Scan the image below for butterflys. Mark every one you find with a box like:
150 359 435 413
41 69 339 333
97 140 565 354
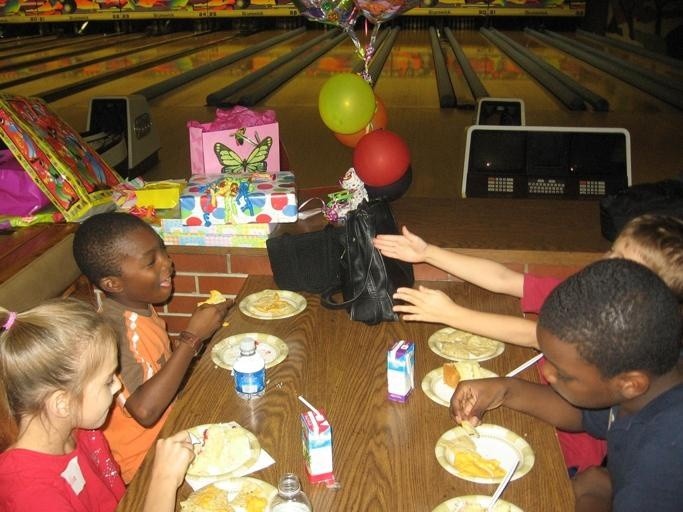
214 136 272 173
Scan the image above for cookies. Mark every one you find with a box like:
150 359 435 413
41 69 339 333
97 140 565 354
461 419 481 438
196 289 226 308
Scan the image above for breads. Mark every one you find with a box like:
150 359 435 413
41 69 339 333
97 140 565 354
187 425 250 477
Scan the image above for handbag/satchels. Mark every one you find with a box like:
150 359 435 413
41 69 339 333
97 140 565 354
343 197 416 327
189 119 282 177
265 225 343 295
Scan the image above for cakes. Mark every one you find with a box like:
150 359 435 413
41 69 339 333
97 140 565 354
444 435 509 478
443 360 480 389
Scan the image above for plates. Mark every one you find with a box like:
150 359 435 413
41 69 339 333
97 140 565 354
239 290 307 320
428 326 506 362
434 423 535 485
421 366 501 410
210 332 289 370
180 423 273 511
430 494 522 512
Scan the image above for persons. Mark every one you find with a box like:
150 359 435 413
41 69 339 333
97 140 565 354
73 212 234 486
0 297 196 512
371 214 683 353
449 258 683 512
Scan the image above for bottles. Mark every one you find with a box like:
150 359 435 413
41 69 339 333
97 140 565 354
233 337 266 422
269 474 310 511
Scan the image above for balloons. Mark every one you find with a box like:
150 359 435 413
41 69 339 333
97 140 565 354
299 0 412 187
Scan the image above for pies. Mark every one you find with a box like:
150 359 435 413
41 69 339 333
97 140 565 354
433 330 497 359
179 479 267 512
247 292 295 316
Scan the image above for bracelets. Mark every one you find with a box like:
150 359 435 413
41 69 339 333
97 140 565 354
175 330 205 356
